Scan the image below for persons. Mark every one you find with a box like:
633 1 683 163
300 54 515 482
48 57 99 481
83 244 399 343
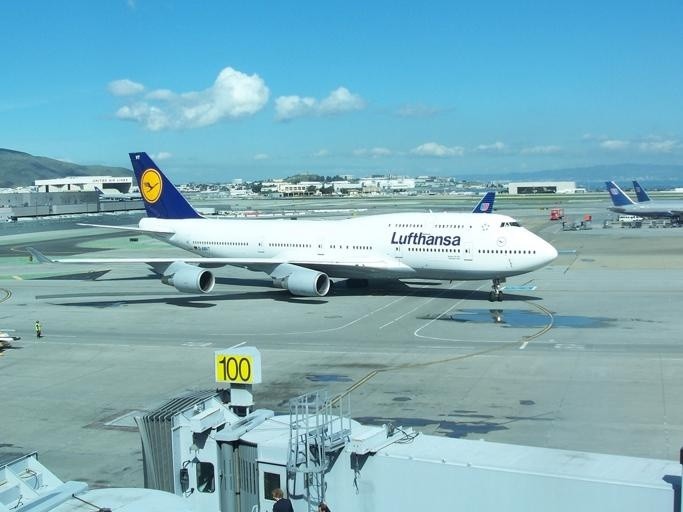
317 501 331 512
271 488 294 512
35 320 41 338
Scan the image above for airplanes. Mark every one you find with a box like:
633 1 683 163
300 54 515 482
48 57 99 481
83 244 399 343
26 153 559 302
604 179 683 226
93 186 142 201
